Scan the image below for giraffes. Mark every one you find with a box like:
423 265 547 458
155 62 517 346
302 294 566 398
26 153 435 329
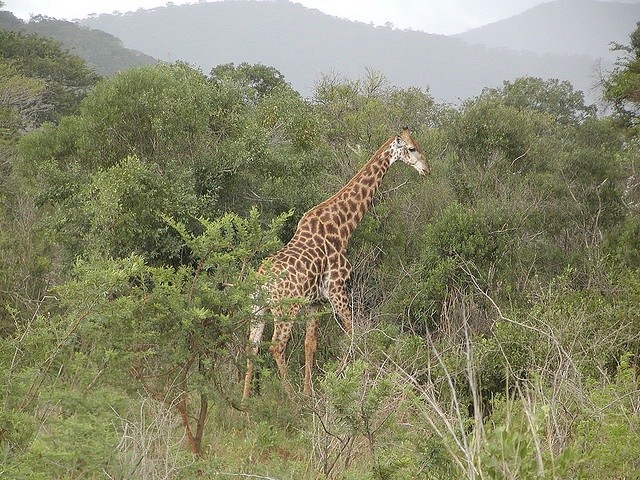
241 124 432 410
